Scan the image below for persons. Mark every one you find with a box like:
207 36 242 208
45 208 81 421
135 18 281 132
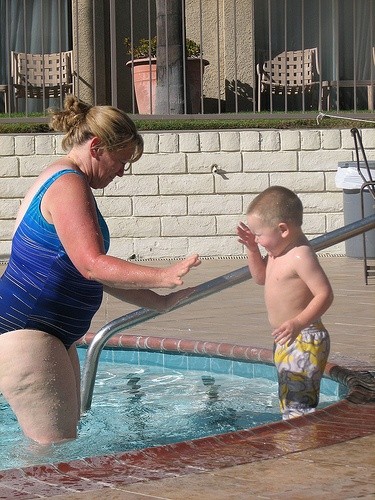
236 186 335 422
0 95 201 445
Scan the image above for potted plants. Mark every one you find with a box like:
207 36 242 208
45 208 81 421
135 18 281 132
124 35 210 114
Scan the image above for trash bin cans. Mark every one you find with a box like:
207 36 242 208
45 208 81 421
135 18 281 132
336 160 375 259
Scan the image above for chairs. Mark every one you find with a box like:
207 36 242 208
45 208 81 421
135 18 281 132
0 50 74 114
255 48 331 111
